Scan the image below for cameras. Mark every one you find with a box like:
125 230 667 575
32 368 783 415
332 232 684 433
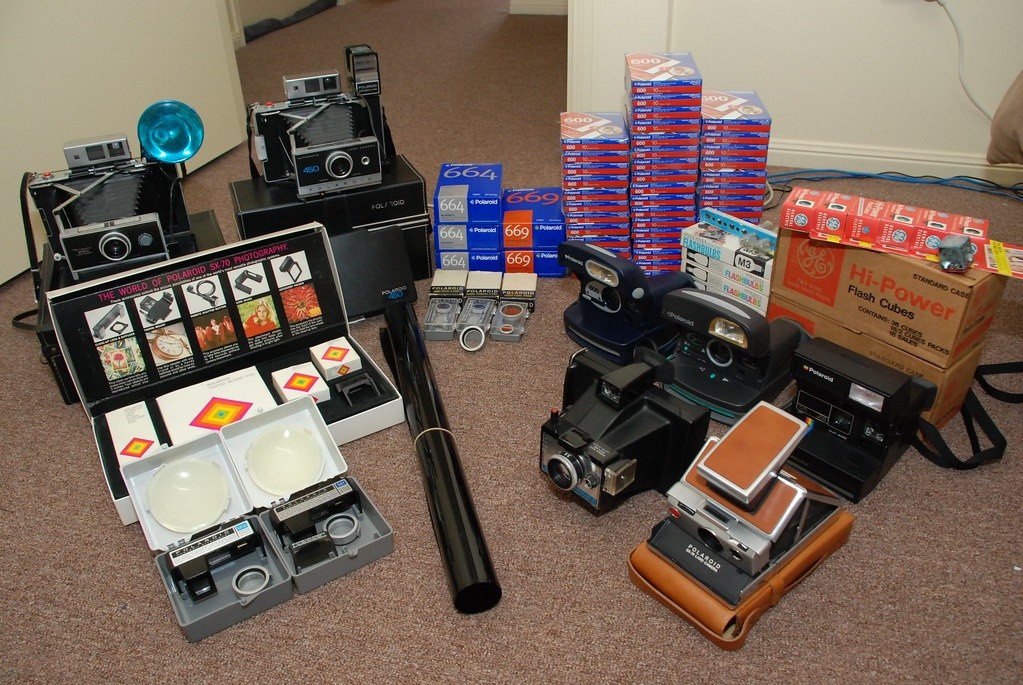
28 99 204 287
537 241 937 653
253 44 395 199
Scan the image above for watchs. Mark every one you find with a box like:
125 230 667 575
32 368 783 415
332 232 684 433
151 328 192 360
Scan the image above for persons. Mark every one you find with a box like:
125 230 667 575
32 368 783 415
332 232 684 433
244 300 278 338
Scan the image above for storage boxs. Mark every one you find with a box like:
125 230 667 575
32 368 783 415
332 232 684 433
44 221 405 525
433 224 500 253
500 249 566 278
778 186 989 263
309 336 363 381
155 365 278 446
428 268 537 315
499 189 565 250
766 292 987 443
270 363 331 405
435 250 501 272
105 400 160 466
770 226 1008 371
559 52 777 317
433 163 502 224
